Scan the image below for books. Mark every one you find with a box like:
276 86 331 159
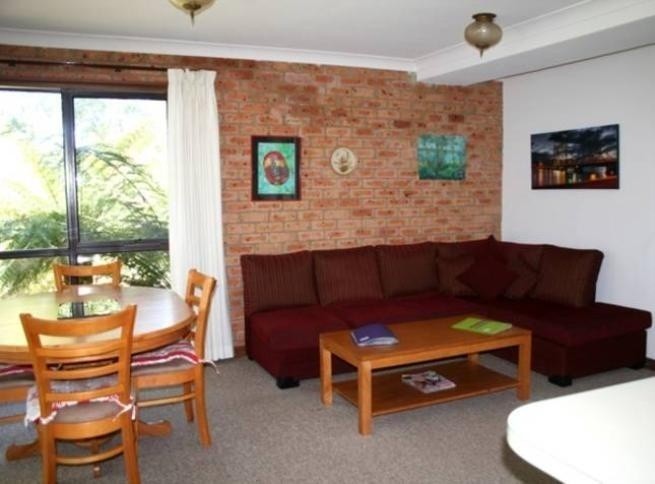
450 316 513 336
351 323 400 347
400 369 457 395
57 298 123 319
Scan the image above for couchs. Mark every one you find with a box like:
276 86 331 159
241 234 653 391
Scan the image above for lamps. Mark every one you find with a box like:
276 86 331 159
464 10 504 56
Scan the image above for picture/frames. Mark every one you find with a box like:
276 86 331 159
249 134 302 203
